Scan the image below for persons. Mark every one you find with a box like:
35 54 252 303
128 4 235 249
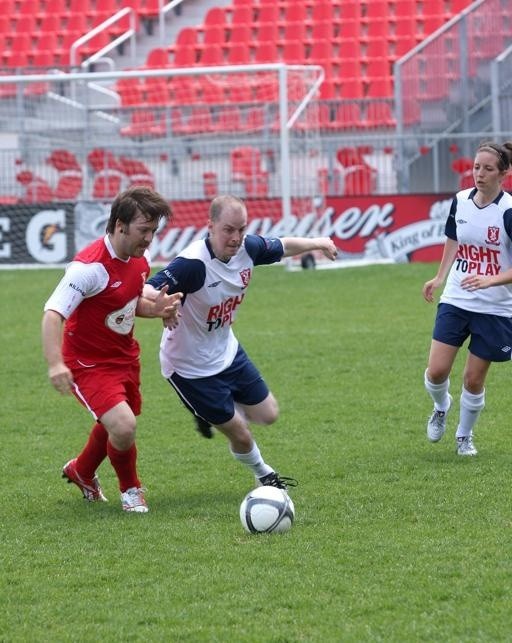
41 187 185 514
146 193 340 488
424 142 512 456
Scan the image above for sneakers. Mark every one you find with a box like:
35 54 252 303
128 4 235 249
456 425 477 456
119 487 148 513
255 466 286 489
195 414 213 439
427 395 454 442
62 459 109 502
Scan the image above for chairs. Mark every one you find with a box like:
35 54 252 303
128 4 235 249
394 1 416 18
19 0 41 20
424 56 449 78
255 21 278 42
0 14 13 38
338 39 361 58
32 50 54 66
365 38 391 58
202 7 228 24
88 30 107 48
330 105 361 128
287 75 303 103
229 23 251 43
309 60 332 79
422 15 444 37
69 0 91 18
365 78 393 98
13 33 32 51
451 1 474 17
8 51 28 67
0 1 17 21
254 41 278 63
361 103 392 128
170 77 198 107
308 40 333 59
421 76 449 101
400 78 420 99
272 106 304 131
96 0 116 16
42 15 61 36
254 72 278 101
189 109 210 132
119 1 142 17
37 33 57 51
45 0 66 20
389 98 423 128
67 13 87 32
423 2 446 16
217 107 242 131
116 79 144 104
16 17 36 39
282 39 306 64
0 33 7 51
201 45 226 66
175 46 195 66
367 17 390 38
245 107 267 133
450 57 475 77
63 34 82 48
311 4 335 20
398 57 419 77
394 37 417 57
306 104 329 129
337 58 361 77
158 109 184 134
228 43 251 63
131 111 155 135
365 57 391 77
140 1 168 17
336 17 361 38
394 16 415 36
146 48 169 67
311 18 335 39
204 24 226 46
176 28 198 45
60 50 80 65
320 80 336 101
364 3 390 19
338 3 362 19
231 5 254 23
338 81 364 99
144 77 169 106
284 5 307 21
199 76 224 104
285 21 306 39
228 73 253 101
258 5 280 22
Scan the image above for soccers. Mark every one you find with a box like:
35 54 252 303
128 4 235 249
239 485 295 535
